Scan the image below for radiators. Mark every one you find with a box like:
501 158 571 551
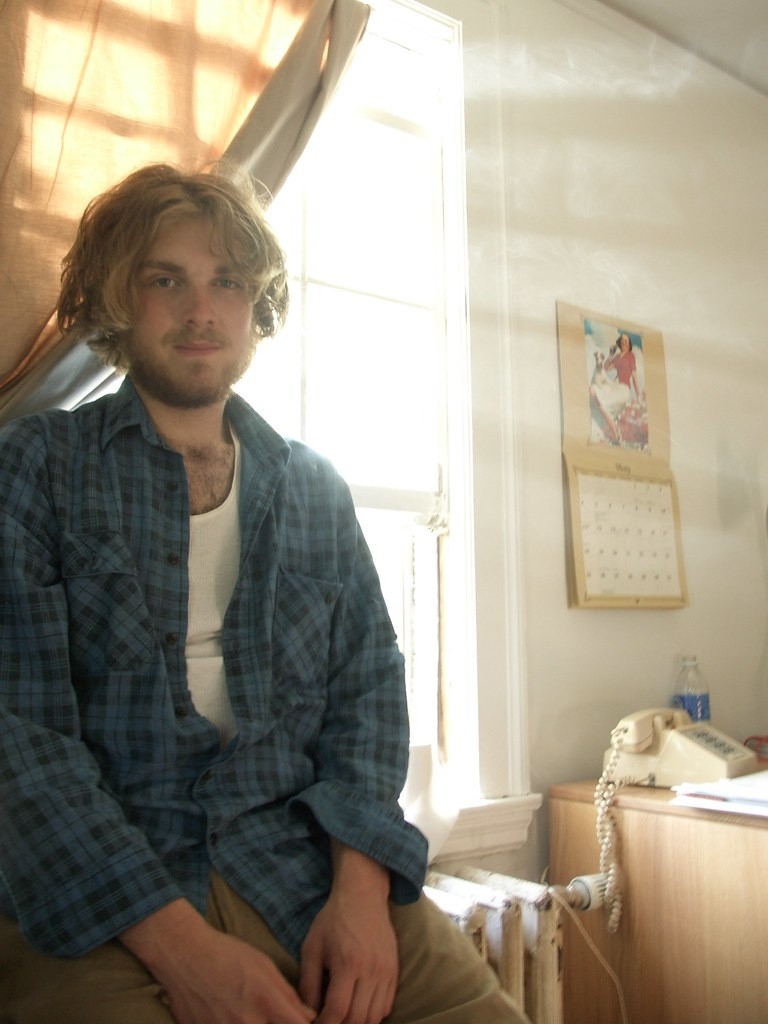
422 865 606 1024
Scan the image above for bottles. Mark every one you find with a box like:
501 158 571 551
674 654 712 724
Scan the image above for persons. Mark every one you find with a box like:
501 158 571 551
0 163 535 1024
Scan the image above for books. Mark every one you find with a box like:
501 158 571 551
670 767 767 820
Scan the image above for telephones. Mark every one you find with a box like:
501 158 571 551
601 706 758 789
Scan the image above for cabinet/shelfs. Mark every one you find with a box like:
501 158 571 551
547 762 768 1024
